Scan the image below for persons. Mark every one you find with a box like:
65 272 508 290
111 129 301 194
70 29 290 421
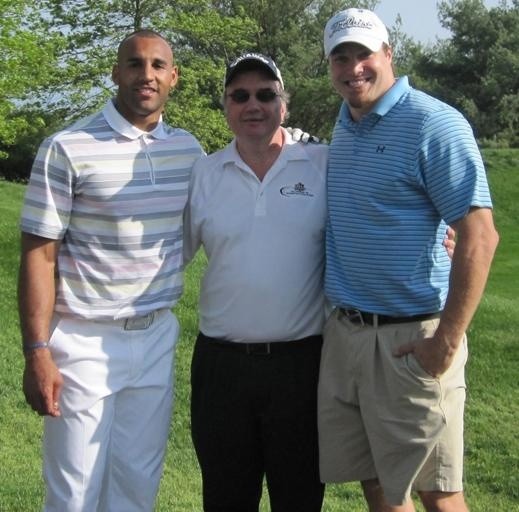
183 53 457 511
318 6 499 512
17 30 319 512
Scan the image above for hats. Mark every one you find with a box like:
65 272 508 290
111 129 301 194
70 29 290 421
322 7 390 64
224 51 285 91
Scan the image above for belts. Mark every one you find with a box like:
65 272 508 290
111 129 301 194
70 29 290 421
199 329 325 365
333 299 450 334
51 305 174 333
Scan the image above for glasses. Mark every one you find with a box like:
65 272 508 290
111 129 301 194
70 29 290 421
224 86 282 105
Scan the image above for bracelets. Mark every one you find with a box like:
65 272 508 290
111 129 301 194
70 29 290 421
22 342 48 352
